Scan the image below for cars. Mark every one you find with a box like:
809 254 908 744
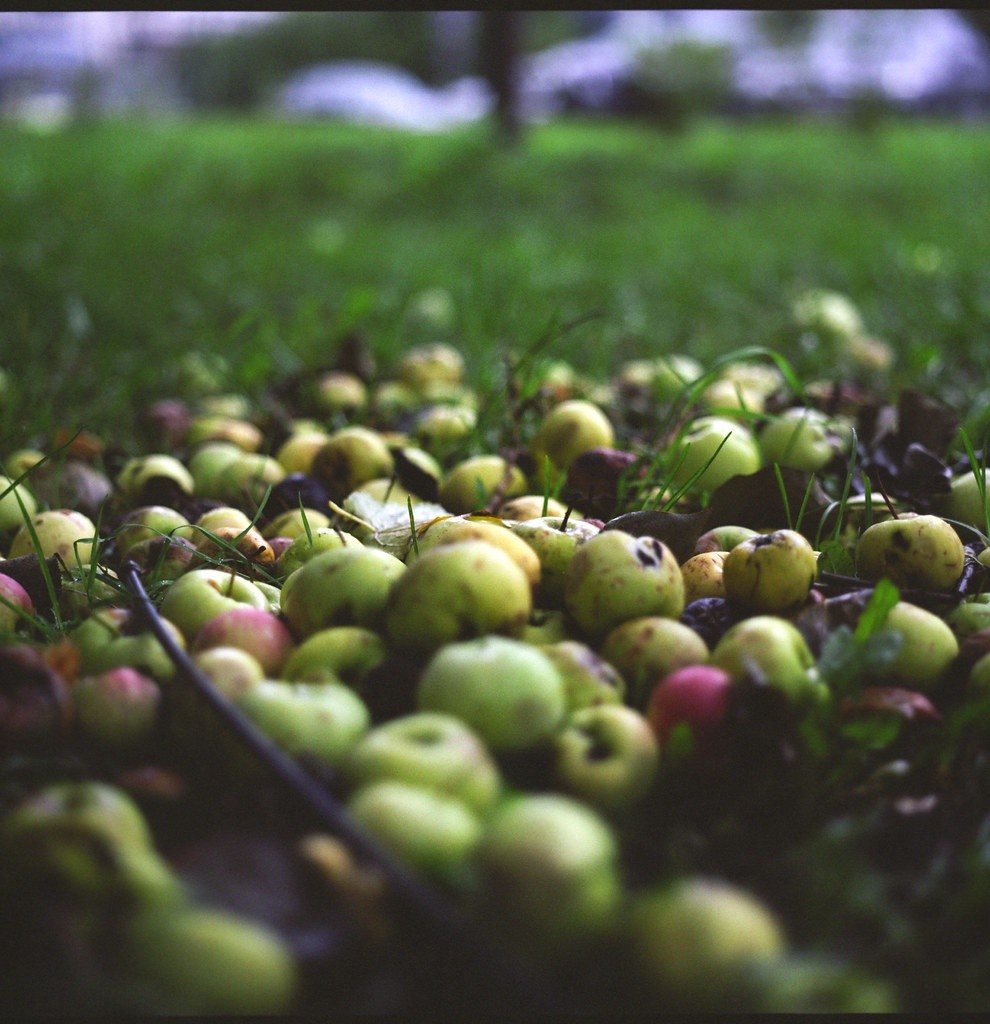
452 10 988 130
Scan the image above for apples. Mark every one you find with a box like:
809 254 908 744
0 285 990 1024
